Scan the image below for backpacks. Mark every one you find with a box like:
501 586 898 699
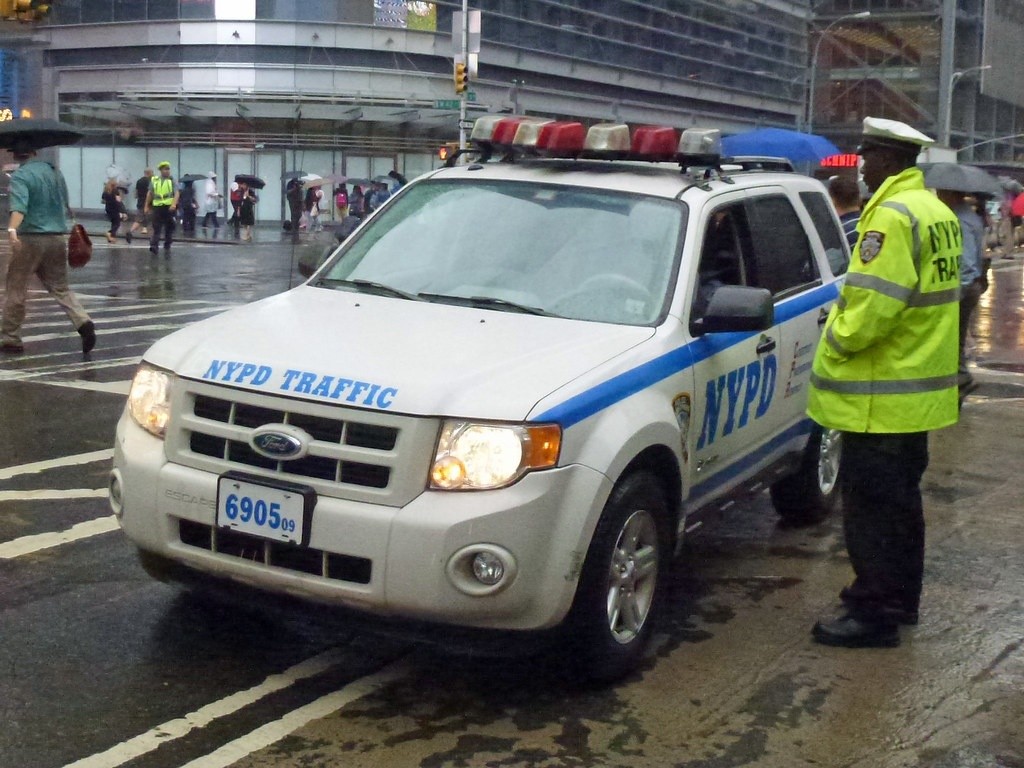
336 193 348 208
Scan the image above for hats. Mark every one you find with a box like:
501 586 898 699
855 116 936 156
157 161 170 169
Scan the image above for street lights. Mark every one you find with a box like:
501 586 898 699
805 11 872 137
943 64 992 149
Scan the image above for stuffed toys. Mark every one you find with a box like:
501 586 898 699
106 162 132 221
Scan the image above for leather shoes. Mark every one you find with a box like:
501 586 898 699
839 585 920 626
811 611 901 648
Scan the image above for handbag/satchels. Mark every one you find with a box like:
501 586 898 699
309 202 320 217
67 225 94 270
980 257 993 294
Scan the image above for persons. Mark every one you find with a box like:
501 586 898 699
805 116 960 644
936 189 984 409
0 139 97 352
825 175 861 251
103 159 405 254
968 192 1024 262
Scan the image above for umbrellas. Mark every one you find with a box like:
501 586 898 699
304 178 334 188
373 175 400 189
721 124 842 162
280 170 308 179
327 175 349 183
301 173 323 179
0 117 83 148
345 178 372 186
234 174 265 189
178 174 208 183
998 176 1023 193
389 170 408 184
914 161 1001 195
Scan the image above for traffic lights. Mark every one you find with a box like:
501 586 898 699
438 143 453 161
454 62 469 94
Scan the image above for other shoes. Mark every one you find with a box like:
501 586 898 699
201 226 223 229
0 342 24 355
77 321 96 353
957 379 978 409
315 225 323 232
184 233 197 238
105 231 117 244
125 229 133 244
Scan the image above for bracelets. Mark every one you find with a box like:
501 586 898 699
8 228 16 231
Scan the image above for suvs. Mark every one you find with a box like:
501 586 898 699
106 112 858 684
